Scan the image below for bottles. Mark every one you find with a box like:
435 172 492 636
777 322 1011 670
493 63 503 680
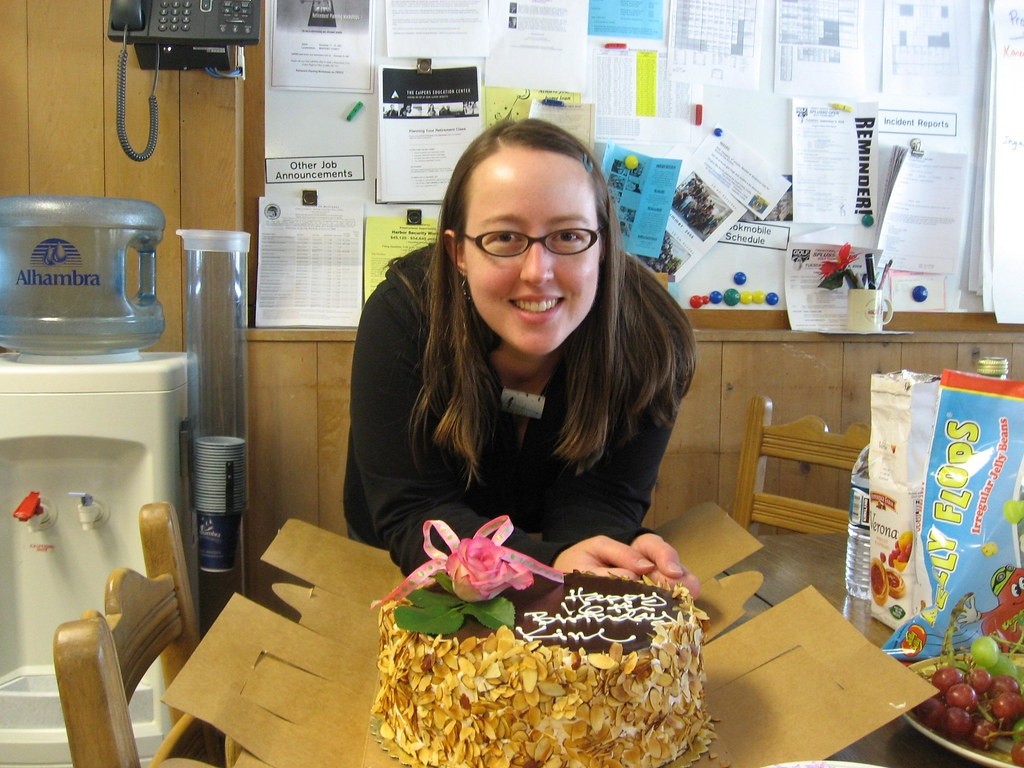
843 444 871 636
0 192 167 354
976 356 1009 380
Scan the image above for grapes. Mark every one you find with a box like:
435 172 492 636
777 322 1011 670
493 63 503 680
912 635 1024 766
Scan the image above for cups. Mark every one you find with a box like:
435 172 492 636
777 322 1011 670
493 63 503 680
192 436 250 573
846 288 894 332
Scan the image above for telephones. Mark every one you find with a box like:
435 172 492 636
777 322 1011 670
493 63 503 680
107 0 260 46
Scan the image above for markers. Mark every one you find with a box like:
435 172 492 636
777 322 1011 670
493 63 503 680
865 253 876 289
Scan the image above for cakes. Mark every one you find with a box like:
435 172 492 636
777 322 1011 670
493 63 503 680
370 569 722 768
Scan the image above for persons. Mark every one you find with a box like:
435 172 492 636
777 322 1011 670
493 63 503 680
672 177 722 236
637 231 678 274
343 119 697 601
387 101 477 118
766 192 793 221
607 159 644 236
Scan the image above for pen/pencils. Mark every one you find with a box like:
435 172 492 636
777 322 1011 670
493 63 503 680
843 258 892 290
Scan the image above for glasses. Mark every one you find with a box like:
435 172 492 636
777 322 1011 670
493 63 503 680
464 227 604 258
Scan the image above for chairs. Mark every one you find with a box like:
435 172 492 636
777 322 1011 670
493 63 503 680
52 497 224 768
729 397 877 539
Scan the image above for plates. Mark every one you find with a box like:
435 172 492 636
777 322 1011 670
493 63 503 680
902 652 1024 768
